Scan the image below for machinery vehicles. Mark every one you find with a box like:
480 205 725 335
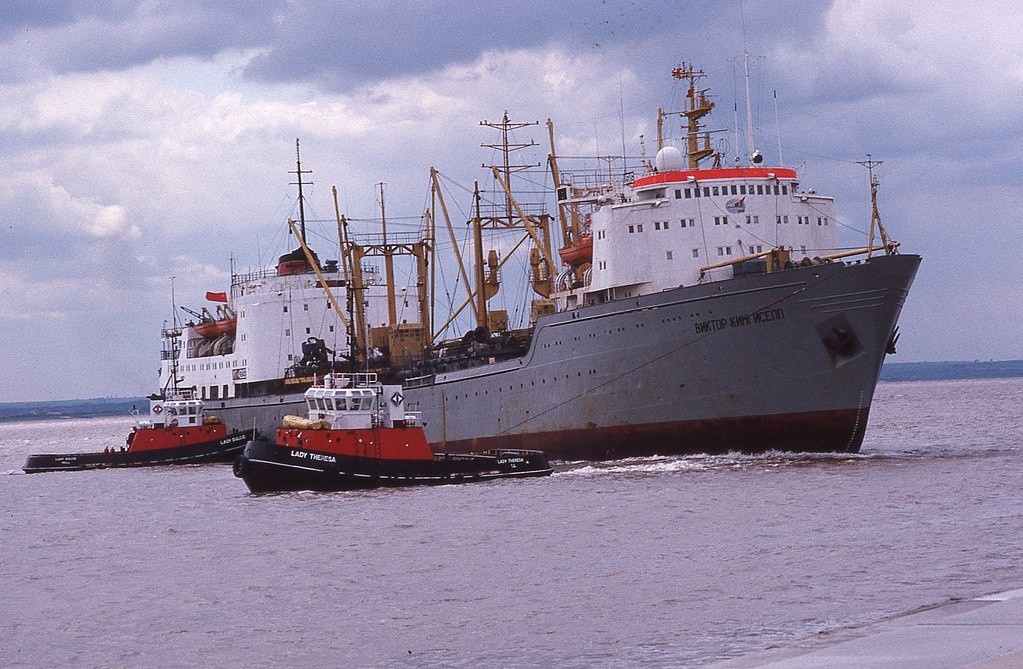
277 119 591 392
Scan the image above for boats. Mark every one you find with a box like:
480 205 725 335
20 389 262 474
230 365 555 496
142 0 923 461
